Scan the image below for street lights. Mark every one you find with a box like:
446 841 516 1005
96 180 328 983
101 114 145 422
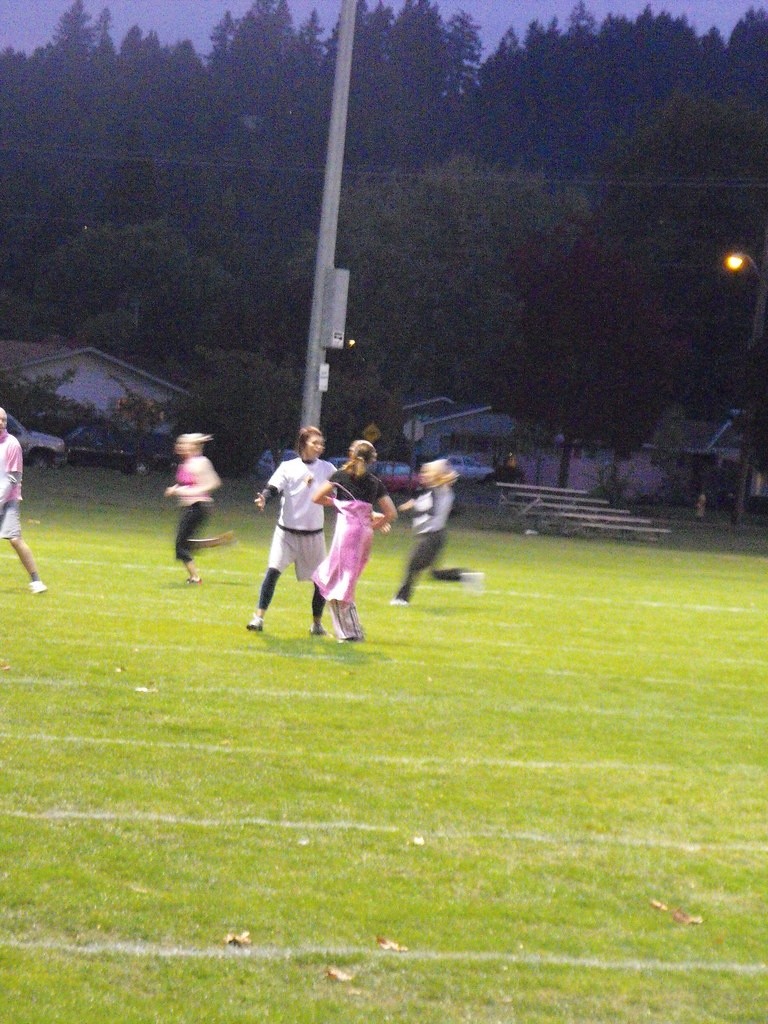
726 250 767 526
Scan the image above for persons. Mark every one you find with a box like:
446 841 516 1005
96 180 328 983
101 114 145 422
0 407 47 595
246 427 390 636
390 460 485 607
311 440 398 642
165 433 237 583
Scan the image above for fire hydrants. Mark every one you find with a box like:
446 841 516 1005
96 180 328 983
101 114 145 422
693 494 709 521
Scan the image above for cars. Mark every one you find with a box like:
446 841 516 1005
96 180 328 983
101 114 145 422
435 454 496 484
375 460 422 492
257 449 300 482
7 414 67 471
66 422 181 477
327 457 350 470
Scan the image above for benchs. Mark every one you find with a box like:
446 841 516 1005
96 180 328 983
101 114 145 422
495 482 672 543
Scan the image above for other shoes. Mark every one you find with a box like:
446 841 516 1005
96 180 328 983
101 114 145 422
29 580 47 594
246 613 264 631
310 623 333 637
390 598 409 606
186 577 202 584
